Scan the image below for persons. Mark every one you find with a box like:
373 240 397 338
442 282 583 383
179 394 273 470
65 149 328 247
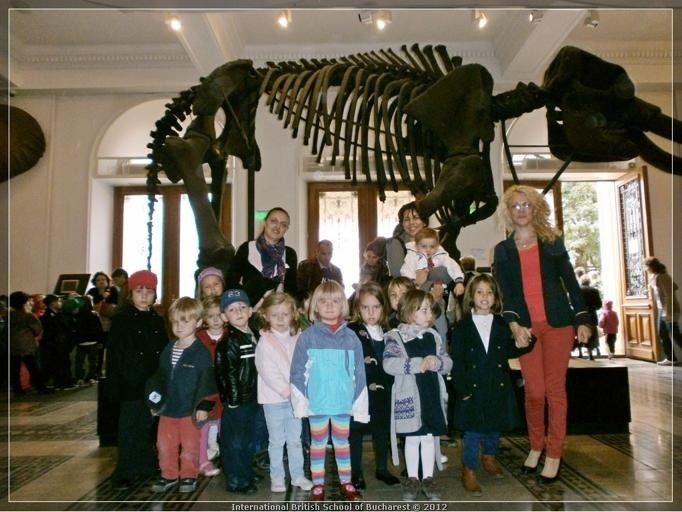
0 184 601 502
642 257 682 366
598 300 619 358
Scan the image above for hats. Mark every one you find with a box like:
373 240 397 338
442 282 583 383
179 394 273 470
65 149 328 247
10 291 94 313
198 266 224 285
220 288 251 313
127 270 157 292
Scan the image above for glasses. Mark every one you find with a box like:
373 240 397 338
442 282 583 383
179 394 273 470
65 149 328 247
508 201 532 210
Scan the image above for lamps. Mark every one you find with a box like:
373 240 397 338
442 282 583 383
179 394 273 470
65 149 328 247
165 13 181 31
278 11 291 30
376 11 391 30
584 11 600 30
472 11 488 29
529 10 544 23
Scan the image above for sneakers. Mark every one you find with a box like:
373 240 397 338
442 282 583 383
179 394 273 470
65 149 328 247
657 358 677 365
23 379 101 395
